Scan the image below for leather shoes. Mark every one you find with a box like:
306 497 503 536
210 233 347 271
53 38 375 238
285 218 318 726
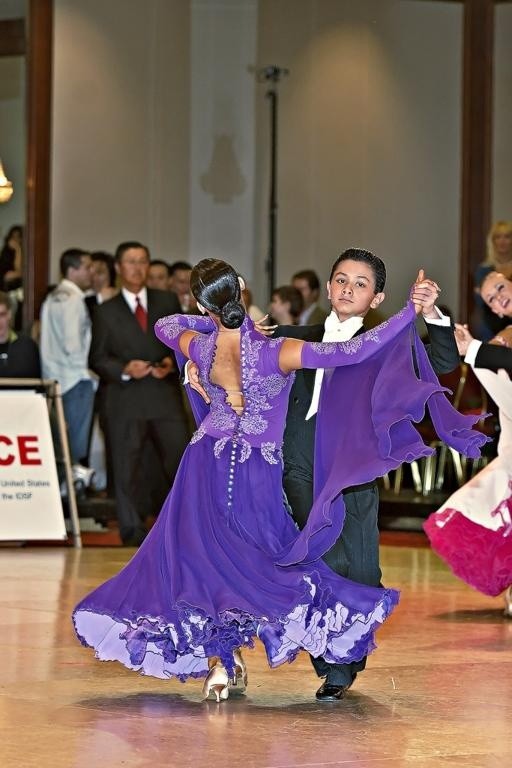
315 664 356 701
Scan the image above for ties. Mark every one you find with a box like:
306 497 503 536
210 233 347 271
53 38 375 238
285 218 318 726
134 297 147 334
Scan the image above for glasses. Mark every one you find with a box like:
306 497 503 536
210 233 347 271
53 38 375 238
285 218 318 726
119 258 151 267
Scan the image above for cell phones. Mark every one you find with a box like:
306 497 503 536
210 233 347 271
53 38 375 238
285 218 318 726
153 361 165 370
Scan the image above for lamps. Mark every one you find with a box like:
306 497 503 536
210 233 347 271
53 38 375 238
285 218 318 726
197 130 246 203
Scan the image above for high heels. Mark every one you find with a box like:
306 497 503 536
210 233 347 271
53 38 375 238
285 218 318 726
228 650 248 694
202 663 229 704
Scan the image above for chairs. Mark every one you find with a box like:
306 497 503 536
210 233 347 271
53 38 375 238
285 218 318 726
370 356 493 499
